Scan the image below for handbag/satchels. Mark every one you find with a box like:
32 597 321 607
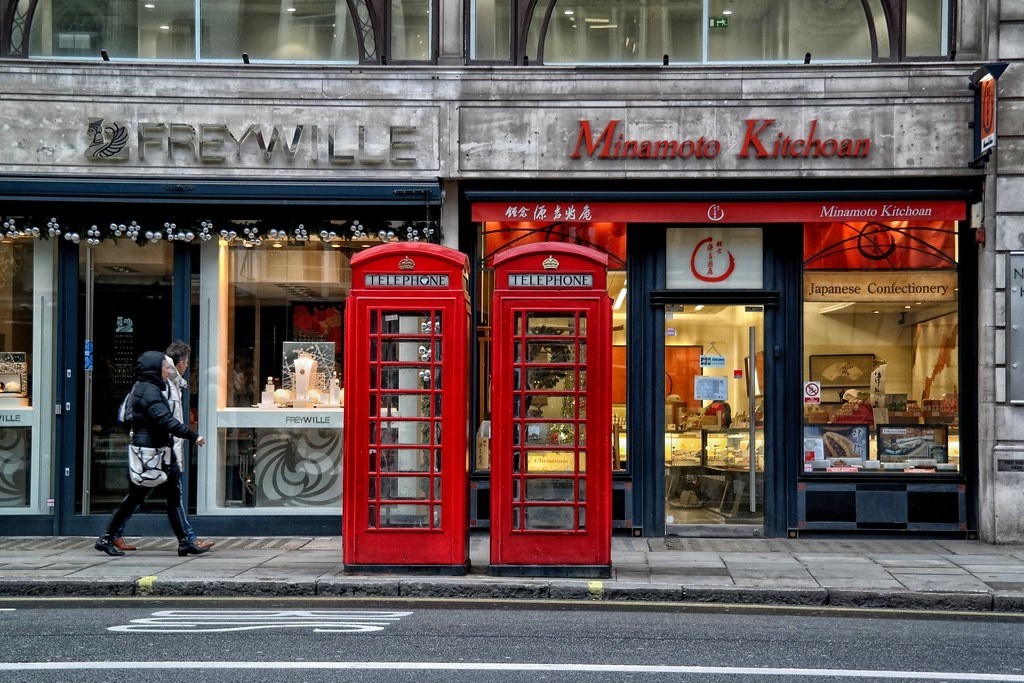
128 444 172 487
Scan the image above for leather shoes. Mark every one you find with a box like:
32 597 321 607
193 539 215 548
112 536 136 550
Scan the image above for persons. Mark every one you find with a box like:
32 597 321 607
704 396 732 429
233 358 253 407
889 434 899 451
96 338 214 557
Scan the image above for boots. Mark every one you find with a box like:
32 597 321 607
167 507 210 556
95 508 128 556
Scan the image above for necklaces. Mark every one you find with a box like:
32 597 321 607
296 357 311 374
